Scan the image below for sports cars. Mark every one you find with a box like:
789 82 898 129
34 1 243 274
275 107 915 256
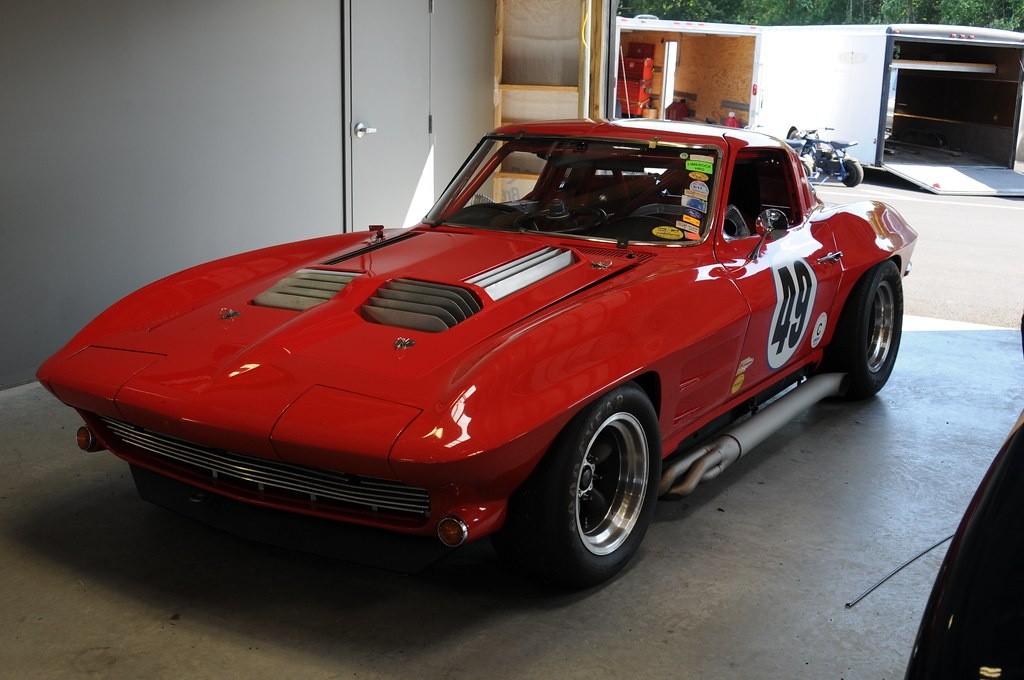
36 117 920 595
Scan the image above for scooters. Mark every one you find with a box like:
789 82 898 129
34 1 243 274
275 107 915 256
740 122 830 187
787 124 864 187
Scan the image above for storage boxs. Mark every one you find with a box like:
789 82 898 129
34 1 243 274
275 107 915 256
616 79 652 115
617 56 654 80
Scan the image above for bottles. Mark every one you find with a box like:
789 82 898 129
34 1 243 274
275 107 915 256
665 97 689 121
725 111 741 128
